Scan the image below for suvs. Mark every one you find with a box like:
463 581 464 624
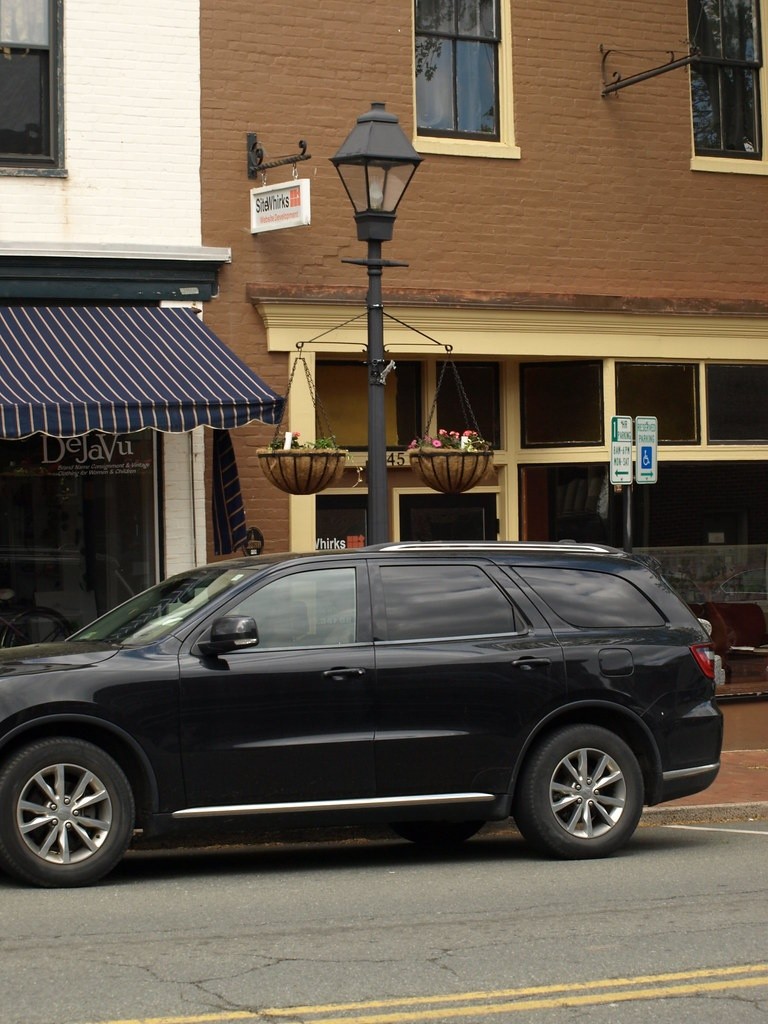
2 536 729 885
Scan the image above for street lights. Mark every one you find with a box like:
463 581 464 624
330 95 426 544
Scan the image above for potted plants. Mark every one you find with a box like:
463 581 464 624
406 448 495 498
255 447 347 497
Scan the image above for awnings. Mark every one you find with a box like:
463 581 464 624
0 303 288 443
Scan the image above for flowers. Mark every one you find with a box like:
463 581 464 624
266 430 342 451
406 429 491 451
